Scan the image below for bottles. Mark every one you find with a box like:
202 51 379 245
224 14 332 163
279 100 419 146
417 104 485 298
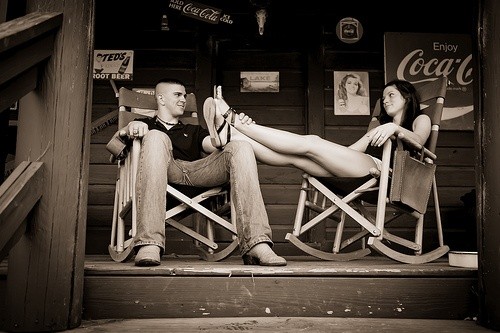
118 55 131 74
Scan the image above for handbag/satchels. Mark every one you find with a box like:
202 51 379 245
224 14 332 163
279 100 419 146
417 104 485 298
393 131 436 216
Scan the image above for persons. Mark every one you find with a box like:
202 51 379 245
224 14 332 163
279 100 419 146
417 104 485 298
112 74 288 267
336 74 369 115
202 79 432 205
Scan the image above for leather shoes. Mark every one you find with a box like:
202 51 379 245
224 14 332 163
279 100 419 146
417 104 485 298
243 242 287 265
133 245 161 266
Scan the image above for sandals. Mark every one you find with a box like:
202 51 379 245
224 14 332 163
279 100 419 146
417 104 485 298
213 85 237 125
203 96 231 148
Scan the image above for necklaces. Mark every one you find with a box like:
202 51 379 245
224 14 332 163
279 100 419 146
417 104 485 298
156 115 179 126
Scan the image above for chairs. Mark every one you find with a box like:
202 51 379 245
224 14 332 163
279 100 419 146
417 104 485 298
285 77 450 264
109 87 239 263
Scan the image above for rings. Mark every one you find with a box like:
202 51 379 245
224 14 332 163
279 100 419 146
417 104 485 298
381 135 385 138
133 129 139 134
